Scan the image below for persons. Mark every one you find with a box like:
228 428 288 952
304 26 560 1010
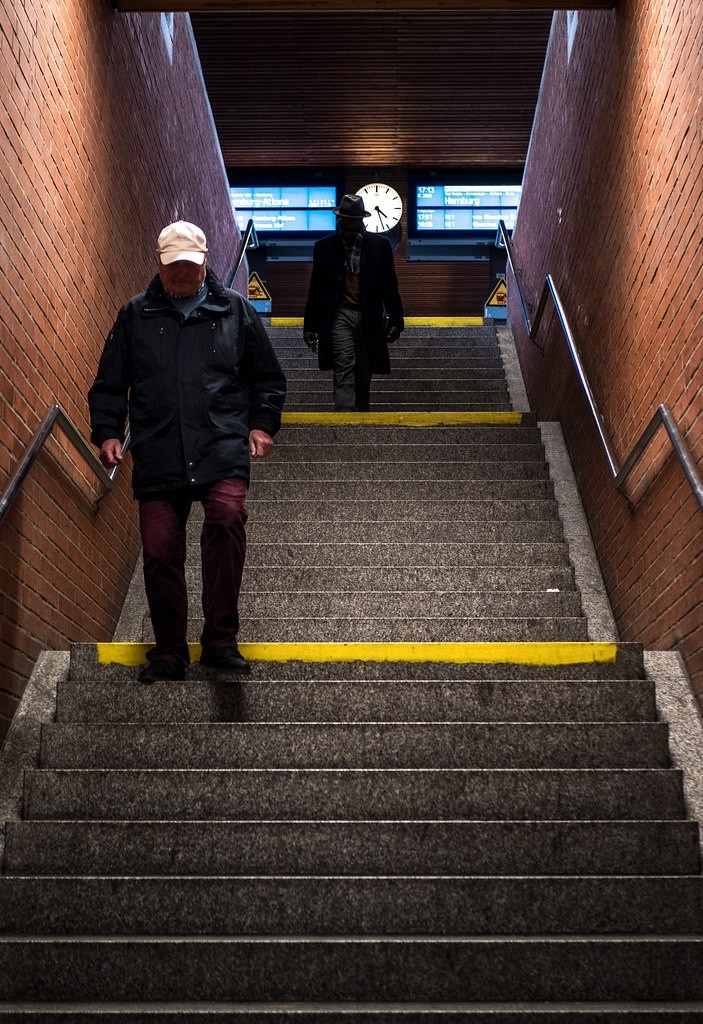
303 195 405 412
88 221 286 682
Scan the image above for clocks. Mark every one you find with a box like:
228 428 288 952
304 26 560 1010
354 182 403 234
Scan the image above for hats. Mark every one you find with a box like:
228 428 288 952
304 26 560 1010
332 195 371 218
157 220 208 265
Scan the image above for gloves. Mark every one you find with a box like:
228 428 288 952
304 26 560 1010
302 331 317 348
386 324 400 343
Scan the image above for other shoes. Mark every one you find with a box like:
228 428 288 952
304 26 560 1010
137 664 185 681
199 645 251 674
